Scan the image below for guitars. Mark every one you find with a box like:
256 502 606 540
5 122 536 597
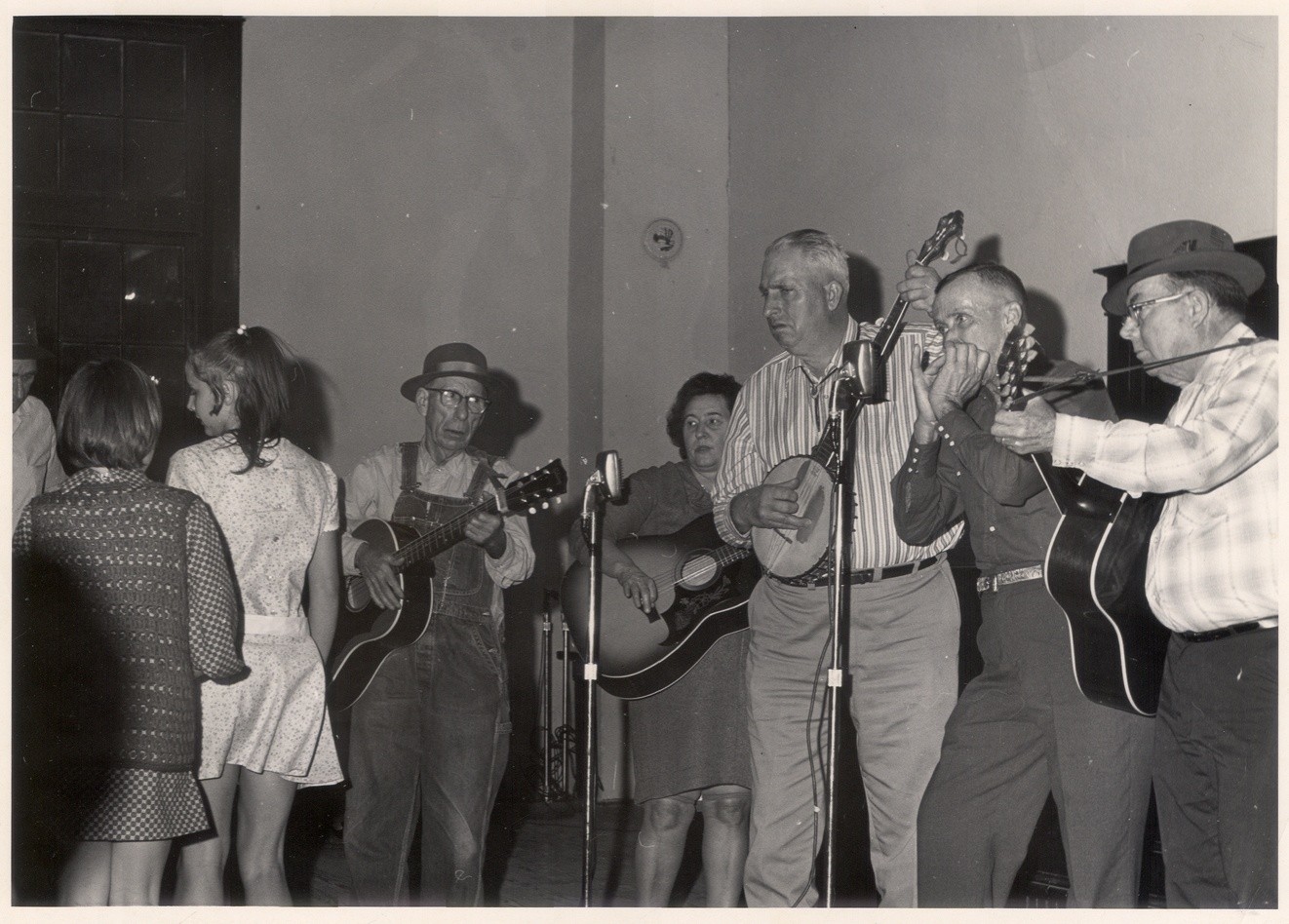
749 210 965 589
301 458 569 713
559 512 762 700
996 321 1165 718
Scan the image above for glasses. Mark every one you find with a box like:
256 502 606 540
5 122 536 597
1119 293 1188 326
425 387 494 415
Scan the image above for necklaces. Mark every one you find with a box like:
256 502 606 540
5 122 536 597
701 484 710 493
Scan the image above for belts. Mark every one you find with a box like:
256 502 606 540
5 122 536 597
761 551 942 588
1175 616 1278 644
976 564 1043 592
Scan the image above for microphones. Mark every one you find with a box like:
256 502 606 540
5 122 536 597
580 450 625 531
829 341 883 426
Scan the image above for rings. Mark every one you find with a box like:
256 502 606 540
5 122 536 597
1001 437 1004 445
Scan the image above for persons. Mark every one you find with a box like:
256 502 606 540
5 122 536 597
989 219 1278 908
888 262 1151 908
11 299 342 906
344 342 532 906
709 227 966 908
563 368 765 908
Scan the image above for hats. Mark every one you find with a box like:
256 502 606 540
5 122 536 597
12 308 58 359
400 342 509 403
1100 220 1267 316
